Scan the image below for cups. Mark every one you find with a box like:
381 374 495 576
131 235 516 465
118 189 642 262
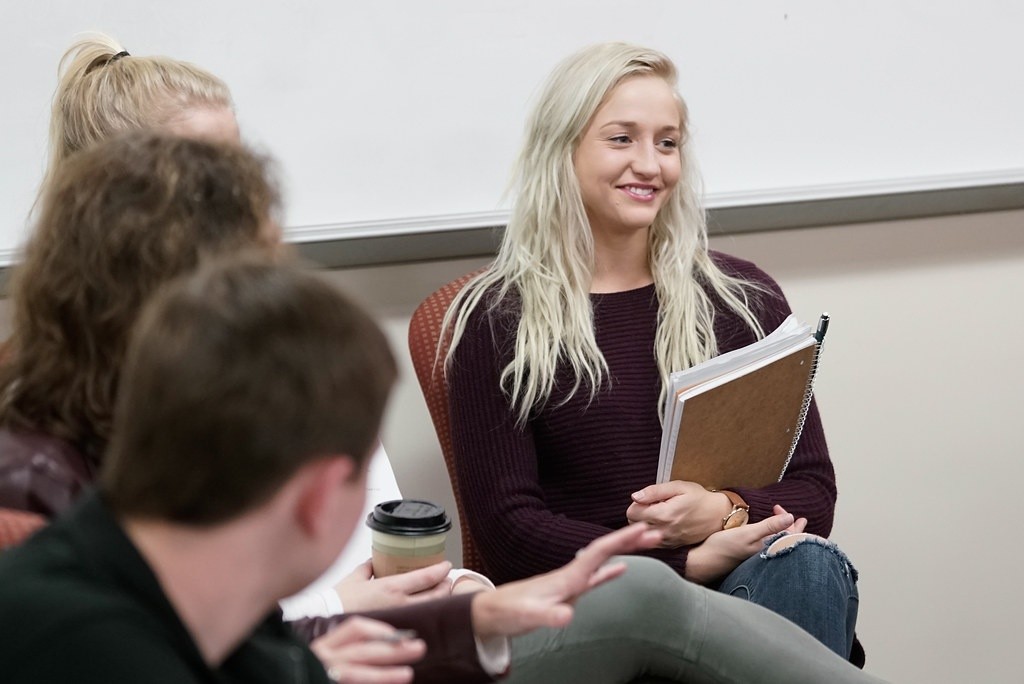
366 500 453 581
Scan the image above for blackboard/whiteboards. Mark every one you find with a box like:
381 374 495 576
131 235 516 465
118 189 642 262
0 0 1024 299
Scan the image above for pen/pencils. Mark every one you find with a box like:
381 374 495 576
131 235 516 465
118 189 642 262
808 311 832 387
371 631 414 642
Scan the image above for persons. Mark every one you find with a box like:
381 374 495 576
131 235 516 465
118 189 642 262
432 41 865 669
0 38 877 684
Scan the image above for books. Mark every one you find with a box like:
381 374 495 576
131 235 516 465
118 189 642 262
657 314 825 492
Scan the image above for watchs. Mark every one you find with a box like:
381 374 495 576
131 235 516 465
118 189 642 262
712 489 750 529
